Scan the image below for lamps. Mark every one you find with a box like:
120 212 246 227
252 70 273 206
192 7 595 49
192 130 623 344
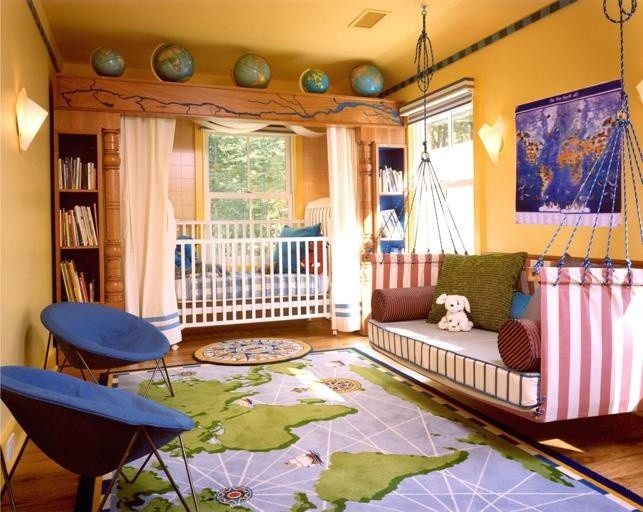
14 86 48 156
476 115 507 164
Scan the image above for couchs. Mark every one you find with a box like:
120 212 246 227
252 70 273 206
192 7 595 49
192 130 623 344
367 250 643 427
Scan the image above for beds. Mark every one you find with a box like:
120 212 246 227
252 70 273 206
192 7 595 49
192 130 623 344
159 193 337 352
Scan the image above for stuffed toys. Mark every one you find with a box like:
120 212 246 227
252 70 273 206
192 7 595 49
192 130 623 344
175 236 197 272
435 293 474 332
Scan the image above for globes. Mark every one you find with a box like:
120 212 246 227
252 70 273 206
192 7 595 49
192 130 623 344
298 67 330 93
150 42 196 84
89 46 126 77
228 51 273 89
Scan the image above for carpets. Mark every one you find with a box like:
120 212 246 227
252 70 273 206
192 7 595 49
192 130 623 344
74 345 643 512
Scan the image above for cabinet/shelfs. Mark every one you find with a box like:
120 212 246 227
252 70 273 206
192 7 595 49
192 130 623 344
51 109 107 371
370 142 409 256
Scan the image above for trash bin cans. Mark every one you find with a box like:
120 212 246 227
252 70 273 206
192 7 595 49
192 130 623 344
348 64 385 98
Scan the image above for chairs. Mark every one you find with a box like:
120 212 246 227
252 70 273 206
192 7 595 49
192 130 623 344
0 361 200 512
37 299 177 399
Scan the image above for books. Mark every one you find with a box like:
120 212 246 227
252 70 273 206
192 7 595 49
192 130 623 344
58 155 99 304
379 165 406 240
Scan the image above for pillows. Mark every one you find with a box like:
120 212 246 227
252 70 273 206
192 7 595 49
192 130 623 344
495 315 543 375
424 251 530 333
370 284 437 324
267 223 323 274
507 291 533 321
521 252 624 324
